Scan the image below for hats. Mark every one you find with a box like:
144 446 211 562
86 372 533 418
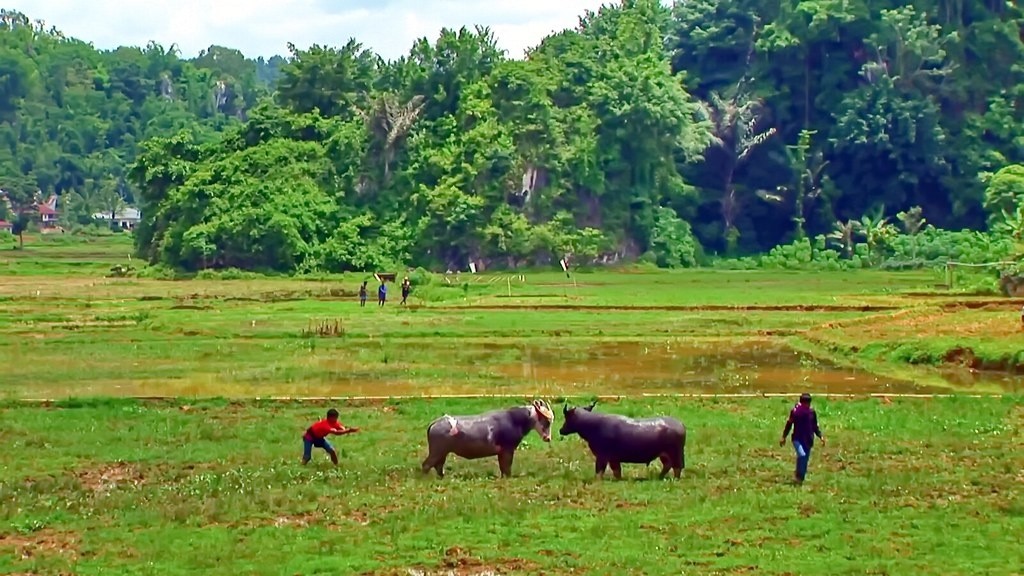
405 277 408 279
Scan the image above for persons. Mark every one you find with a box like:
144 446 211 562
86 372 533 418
359 280 369 307
378 280 387 306
780 393 825 484
400 276 410 305
300 409 360 466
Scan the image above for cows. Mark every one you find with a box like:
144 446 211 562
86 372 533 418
423 399 553 479
559 399 685 482
